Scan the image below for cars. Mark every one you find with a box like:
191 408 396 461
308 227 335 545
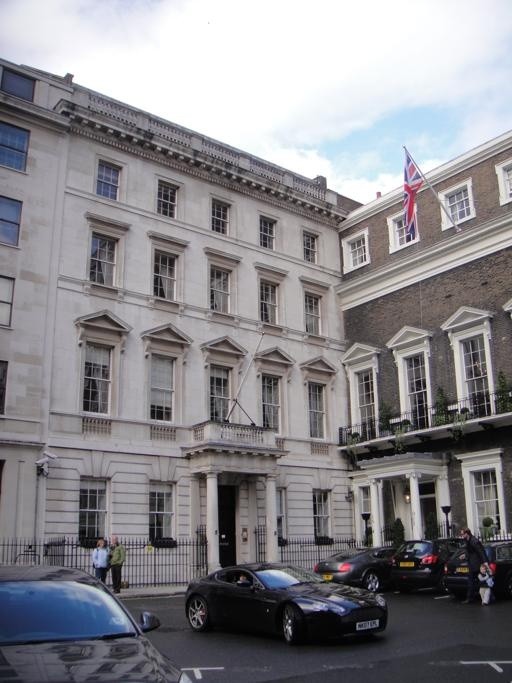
309 542 398 590
444 537 512 599
0 559 195 682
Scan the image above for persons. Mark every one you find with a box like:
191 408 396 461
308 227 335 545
475 561 495 605
391 517 407 546
458 525 495 605
109 535 126 593
234 571 250 584
92 539 112 583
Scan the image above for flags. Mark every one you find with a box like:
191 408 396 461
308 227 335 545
401 150 425 240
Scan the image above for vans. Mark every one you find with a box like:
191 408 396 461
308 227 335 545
389 537 468 593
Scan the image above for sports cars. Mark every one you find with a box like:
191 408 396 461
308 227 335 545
177 560 391 649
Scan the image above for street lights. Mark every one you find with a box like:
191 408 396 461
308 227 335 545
360 511 373 546
439 503 453 538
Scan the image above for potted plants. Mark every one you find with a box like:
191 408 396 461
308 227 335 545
376 396 394 437
477 517 497 539
458 406 473 420
399 418 416 432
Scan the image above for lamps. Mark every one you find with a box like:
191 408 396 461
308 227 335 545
401 479 413 506
